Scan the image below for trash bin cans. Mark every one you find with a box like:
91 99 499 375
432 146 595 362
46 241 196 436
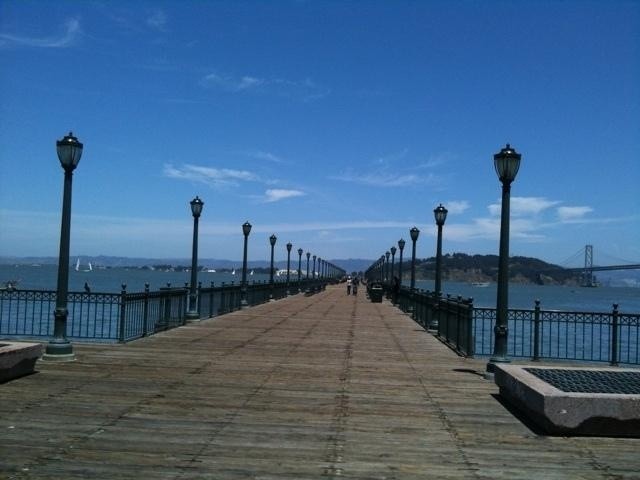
371 285 384 302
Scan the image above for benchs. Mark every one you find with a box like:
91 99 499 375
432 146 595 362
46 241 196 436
306 282 326 295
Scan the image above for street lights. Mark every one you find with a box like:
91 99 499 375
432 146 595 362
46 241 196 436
286 242 292 295
45 132 83 354
298 248 303 291
268 235 277 299
185 196 204 320
432 204 448 330
396 238 405 304
488 144 521 373
241 221 252 306
365 247 396 299
407 226 420 312
306 253 346 290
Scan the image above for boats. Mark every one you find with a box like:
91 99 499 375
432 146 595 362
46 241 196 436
473 283 488 287
208 270 216 272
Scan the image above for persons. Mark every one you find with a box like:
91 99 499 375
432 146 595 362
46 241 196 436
346 275 352 295
392 277 399 303
383 275 392 299
352 277 359 296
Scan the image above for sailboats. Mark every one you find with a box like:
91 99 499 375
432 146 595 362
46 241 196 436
250 270 254 275
75 258 93 272
232 270 236 275
